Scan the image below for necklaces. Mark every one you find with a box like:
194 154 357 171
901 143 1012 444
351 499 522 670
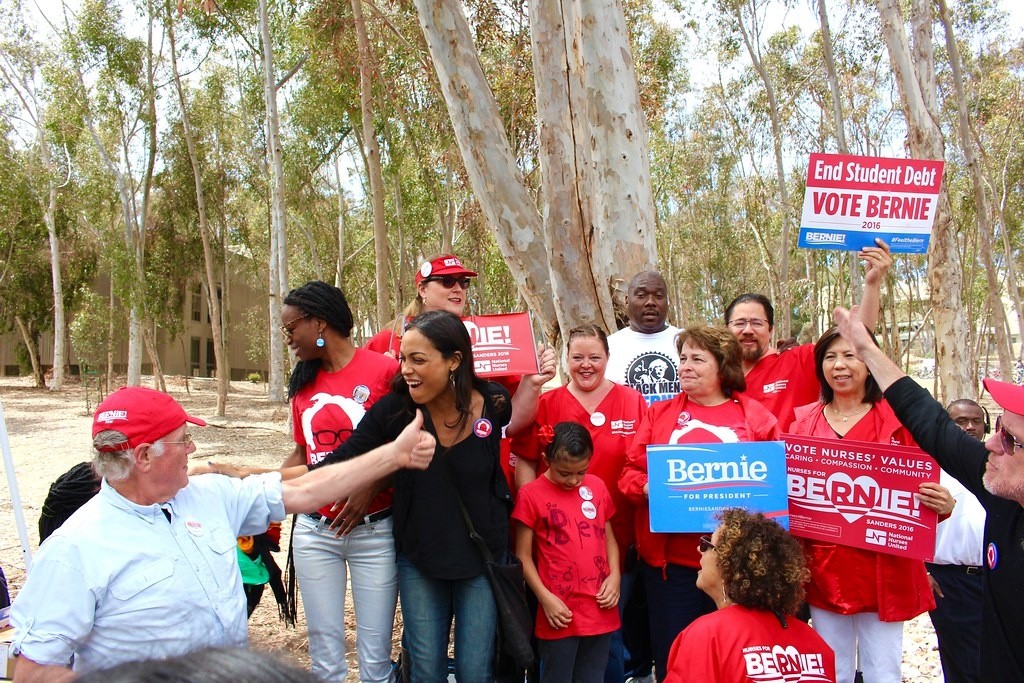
827 403 871 422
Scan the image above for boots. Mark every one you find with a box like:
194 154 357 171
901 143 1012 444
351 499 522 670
401 639 413 683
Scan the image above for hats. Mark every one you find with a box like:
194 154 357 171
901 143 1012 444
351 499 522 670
414 256 477 286
92 385 207 453
982 379 1024 416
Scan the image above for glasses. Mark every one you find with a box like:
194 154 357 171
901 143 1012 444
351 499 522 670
727 318 769 329
698 535 716 552
425 273 471 290
278 312 311 337
157 432 193 448
995 413 1024 456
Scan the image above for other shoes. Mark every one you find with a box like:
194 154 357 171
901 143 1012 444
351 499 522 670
625 670 655 683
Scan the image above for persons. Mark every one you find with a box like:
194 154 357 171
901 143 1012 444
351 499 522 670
10 385 437 683
785 326 955 683
508 422 621 683
725 238 893 432
501 324 646 683
616 335 781 683
661 506 836 683
601 271 686 407
833 303 1024 683
190 310 513 683
923 398 982 683
71 643 322 683
362 253 556 435
276 280 399 683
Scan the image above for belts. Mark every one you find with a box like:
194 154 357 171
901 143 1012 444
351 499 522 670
308 507 391 526
943 561 985 576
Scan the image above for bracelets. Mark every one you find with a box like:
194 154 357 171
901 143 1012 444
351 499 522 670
927 572 930 577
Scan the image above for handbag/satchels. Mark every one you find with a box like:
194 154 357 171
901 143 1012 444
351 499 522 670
483 557 537 667
